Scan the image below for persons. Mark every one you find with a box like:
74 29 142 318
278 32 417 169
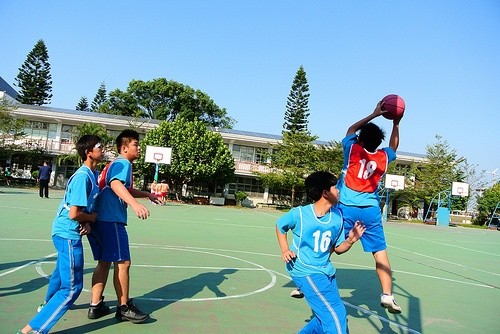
290 94 405 314
86 129 162 324
274 169 367 334
38 160 52 198
16 134 106 334
150 179 169 206
4 168 11 186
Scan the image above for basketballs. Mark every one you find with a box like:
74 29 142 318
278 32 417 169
380 93 405 120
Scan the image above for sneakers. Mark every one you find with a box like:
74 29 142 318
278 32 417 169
115 298 149 324
37 301 45 313
15 329 43 334
87 296 110 320
290 288 305 299
380 293 403 314
